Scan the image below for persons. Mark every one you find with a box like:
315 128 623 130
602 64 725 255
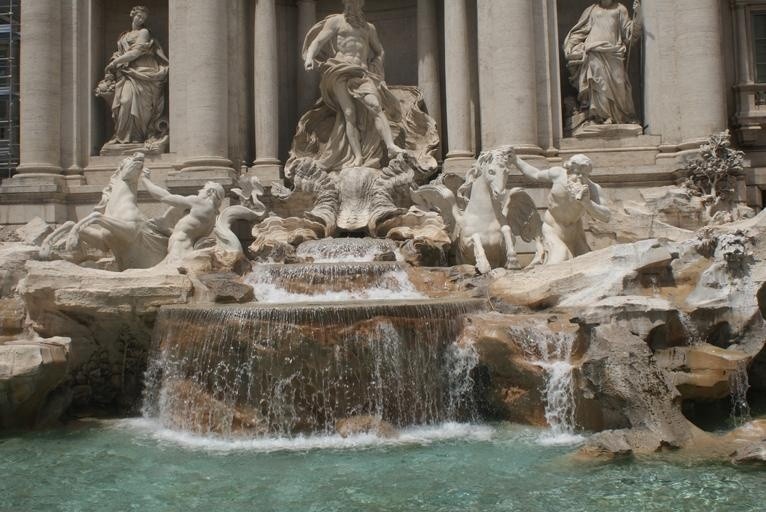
140 167 225 254
105 5 169 143
304 0 405 167
512 154 611 265
562 0 642 123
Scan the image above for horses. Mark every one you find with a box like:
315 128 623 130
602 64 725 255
453 147 522 277
39 150 170 270
